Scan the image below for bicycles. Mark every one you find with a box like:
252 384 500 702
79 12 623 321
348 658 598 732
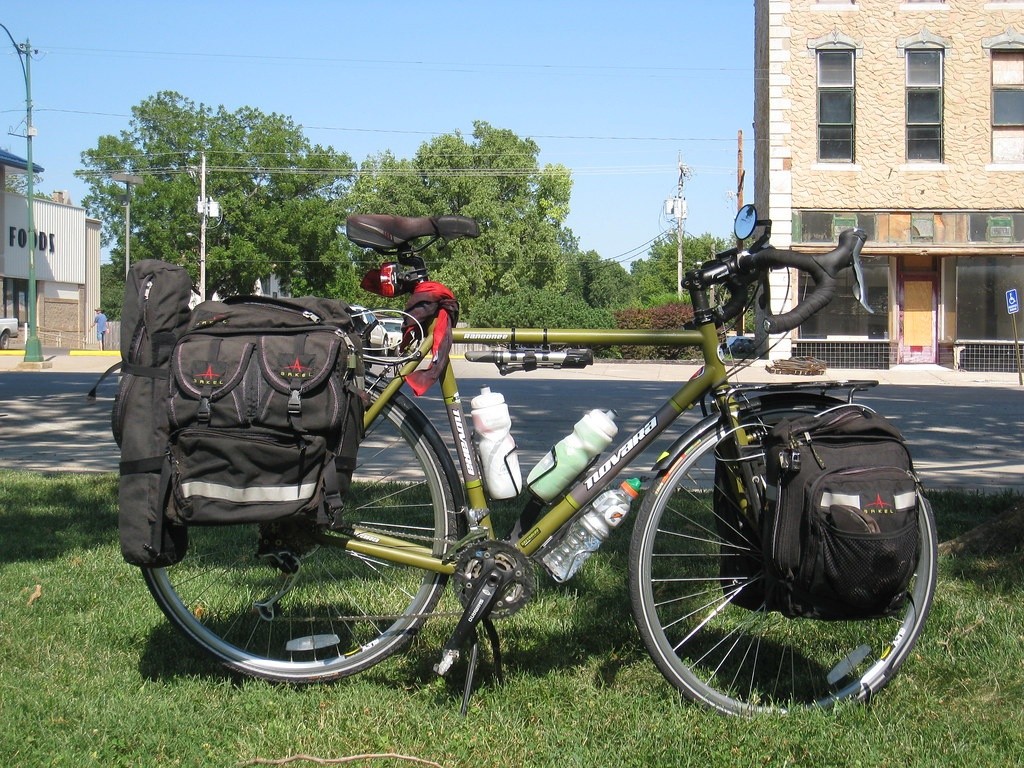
141 203 939 719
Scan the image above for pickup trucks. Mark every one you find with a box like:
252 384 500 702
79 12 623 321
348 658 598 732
0 318 19 349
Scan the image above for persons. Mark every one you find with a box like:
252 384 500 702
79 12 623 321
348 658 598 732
91 308 110 351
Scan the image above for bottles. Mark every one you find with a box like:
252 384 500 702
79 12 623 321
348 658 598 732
538 477 642 582
526 407 619 503
471 383 522 499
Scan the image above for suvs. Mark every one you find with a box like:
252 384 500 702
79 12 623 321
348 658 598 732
379 318 417 357
350 306 389 356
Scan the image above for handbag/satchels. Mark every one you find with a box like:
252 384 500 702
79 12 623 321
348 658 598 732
764 401 924 623
715 410 781 608
169 291 371 528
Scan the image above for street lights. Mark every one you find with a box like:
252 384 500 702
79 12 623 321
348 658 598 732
187 165 206 302
113 174 143 279
